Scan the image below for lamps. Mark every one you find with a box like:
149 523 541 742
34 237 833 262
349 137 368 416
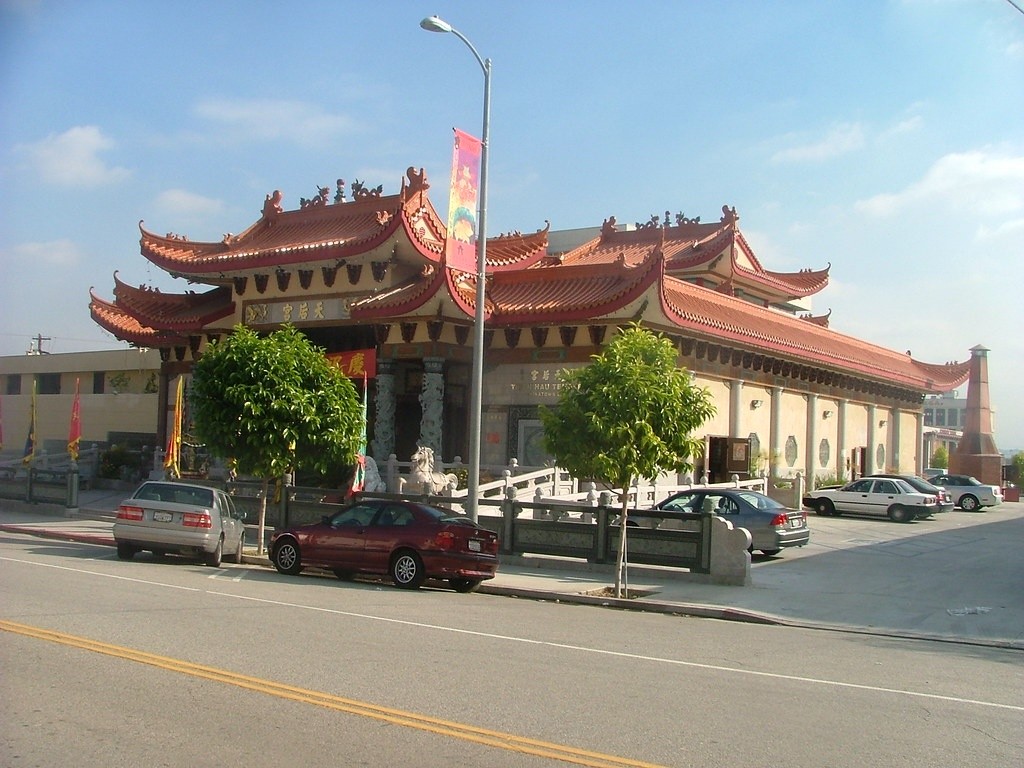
752 400 763 407
880 420 887 427
824 411 834 418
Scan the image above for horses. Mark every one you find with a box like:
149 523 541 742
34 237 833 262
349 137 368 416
405 445 458 495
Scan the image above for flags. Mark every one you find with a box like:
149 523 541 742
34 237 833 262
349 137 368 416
0 393 3 449
344 370 367 500
162 373 182 479
68 377 82 462
22 380 37 466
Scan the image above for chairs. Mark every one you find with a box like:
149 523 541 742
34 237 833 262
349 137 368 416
141 492 160 500
717 498 726 513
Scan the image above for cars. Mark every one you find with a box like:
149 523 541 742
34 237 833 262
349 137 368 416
268 500 500 594
803 476 937 525
925 474 1004 512
816 475 955 519
613 486 809 556
113 481 246 568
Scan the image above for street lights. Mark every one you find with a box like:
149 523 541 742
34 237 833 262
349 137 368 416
420 13 492 523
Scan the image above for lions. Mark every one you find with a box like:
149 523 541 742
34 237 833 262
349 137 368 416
364 456 386 493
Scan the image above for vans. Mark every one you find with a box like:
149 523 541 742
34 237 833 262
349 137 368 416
924 468 948 482
